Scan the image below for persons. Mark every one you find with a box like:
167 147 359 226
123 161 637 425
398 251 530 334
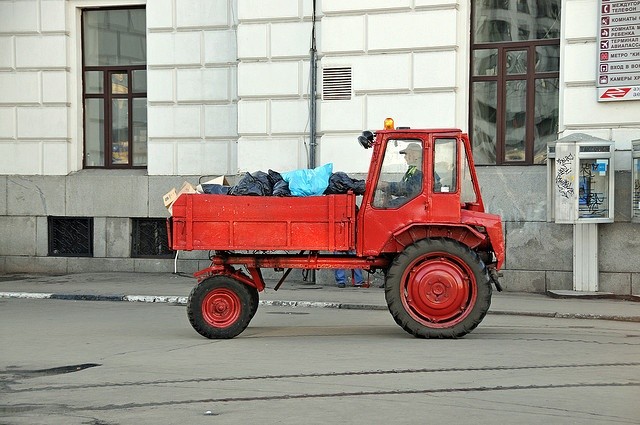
376 143 442 206
333 204 368 288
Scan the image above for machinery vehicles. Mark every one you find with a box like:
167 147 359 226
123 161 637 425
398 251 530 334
166 118 506 339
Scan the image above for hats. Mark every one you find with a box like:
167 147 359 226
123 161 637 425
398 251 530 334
399 143 422 153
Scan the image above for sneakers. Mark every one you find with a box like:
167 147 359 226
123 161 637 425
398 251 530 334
338 283 345 288
355 282 369 287
